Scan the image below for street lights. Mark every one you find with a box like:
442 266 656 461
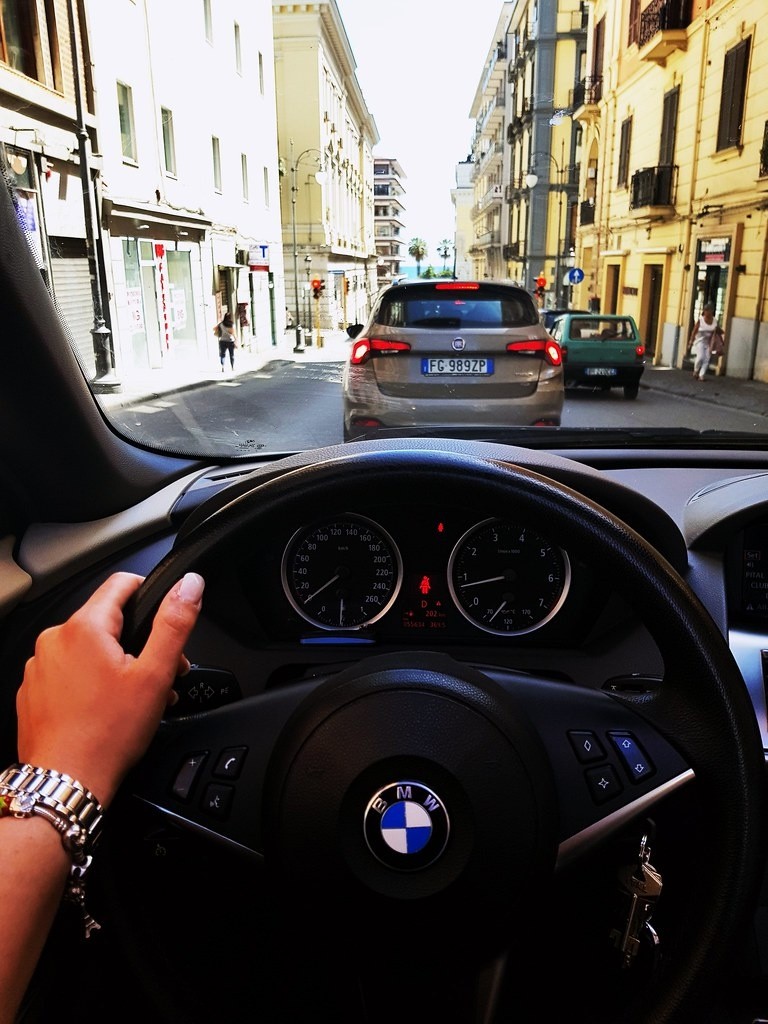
526 149 563 310
291 148 329 353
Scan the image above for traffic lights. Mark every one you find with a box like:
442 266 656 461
344 277 350 294
312 280 320 299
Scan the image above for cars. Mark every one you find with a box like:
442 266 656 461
548 314 645 400
537 308 596 337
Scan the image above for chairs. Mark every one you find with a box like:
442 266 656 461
464 303 501 325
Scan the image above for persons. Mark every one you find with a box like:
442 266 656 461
286 307 294 335
687 304 718 381
0 574 205 1019
214 313 237 372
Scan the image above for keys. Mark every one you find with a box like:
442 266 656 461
620 839 664 969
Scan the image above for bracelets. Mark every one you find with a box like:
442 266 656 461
0 763 106 939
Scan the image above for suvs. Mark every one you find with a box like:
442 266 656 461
341 275 565 443
